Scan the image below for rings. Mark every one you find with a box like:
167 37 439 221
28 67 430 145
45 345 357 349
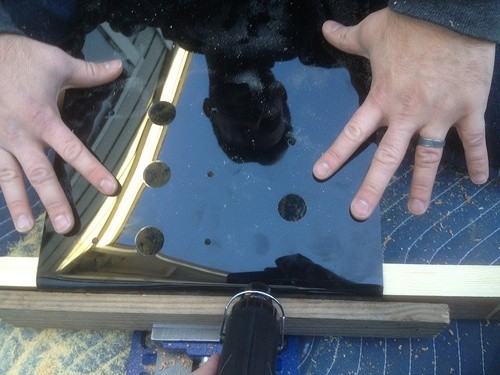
417 138 446 148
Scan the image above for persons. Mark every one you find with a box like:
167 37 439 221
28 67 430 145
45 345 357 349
0 0 499 236
188 353 219 375
8 0 367 166
